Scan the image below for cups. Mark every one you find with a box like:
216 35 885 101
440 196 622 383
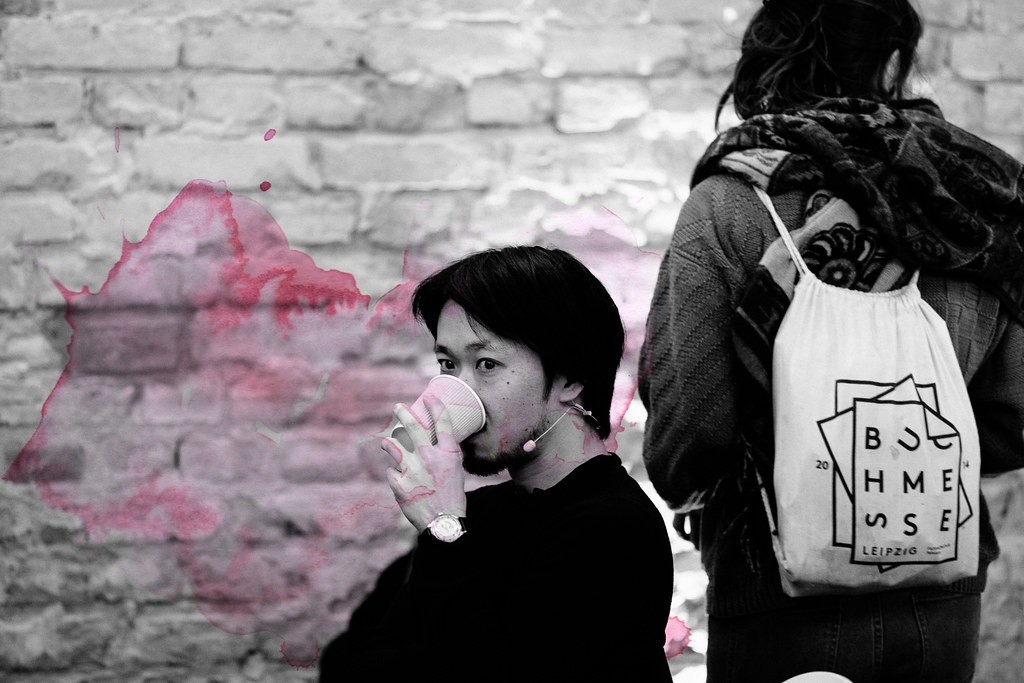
390 374 486 454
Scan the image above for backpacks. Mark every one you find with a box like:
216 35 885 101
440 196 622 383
751 187 980 595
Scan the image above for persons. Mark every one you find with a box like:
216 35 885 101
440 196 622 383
637 0 1024 683
319 245 679 683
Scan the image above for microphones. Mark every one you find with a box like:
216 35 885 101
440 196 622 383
523 408 570 452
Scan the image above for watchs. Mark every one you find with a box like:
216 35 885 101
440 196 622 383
414 509 473 545
